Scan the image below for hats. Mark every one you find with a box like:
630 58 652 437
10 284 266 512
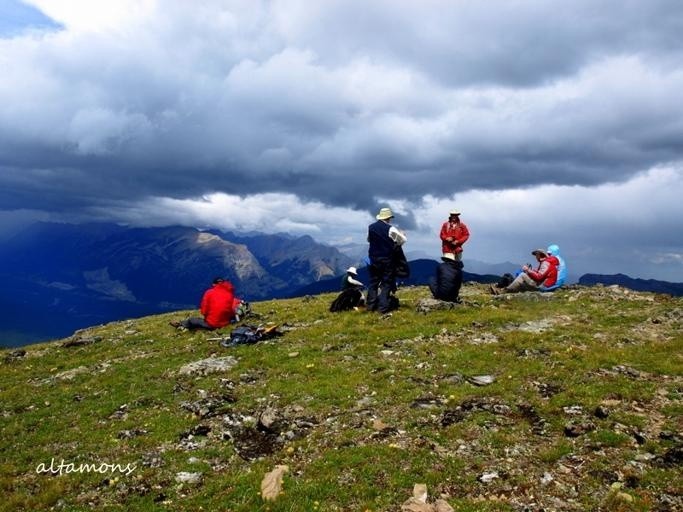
346 266 357 275
375 208 393 220
212 277 224 284
440 253 456 261
532 248 549 258
449 207 461 215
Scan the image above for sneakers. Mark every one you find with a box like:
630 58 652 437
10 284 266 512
170 320 181 328
490 285 501 295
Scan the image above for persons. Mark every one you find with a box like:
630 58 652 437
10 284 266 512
365 208 406 313
438 208 469 262
539 243 568 291
491 249 559 295
340 266 364 291
168 277 240 331
428 252 462 303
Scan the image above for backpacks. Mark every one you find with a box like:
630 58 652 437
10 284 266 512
330 289 360 312
220 323 278 347
498 273 514 288
232 297 251 322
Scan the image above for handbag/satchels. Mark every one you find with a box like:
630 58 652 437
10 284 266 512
394 245 409 277
377 295 399 312
